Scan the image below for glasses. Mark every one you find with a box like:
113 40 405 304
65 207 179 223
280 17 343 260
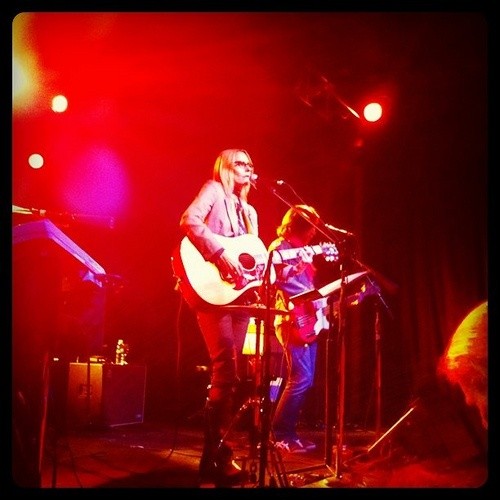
234 161 255 167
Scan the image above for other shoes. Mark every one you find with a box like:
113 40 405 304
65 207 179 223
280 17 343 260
199 463 223 478
223 460 250 481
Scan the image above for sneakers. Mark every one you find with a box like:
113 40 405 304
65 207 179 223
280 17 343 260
270 436 316 453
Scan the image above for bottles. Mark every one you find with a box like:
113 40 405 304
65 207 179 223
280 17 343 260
115 339 124 365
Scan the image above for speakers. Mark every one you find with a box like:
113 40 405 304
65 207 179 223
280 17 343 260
68 362 148 428
366 399 487 472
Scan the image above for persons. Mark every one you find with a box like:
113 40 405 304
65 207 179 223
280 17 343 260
444 300 489 428
268 204 329 453
180 149 314 485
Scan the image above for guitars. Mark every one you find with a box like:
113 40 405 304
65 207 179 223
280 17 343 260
286 282 380 341
171 230 339 311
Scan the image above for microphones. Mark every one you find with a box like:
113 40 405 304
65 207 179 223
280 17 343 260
250 173 285 186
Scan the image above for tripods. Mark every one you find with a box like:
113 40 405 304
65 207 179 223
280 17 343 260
215 269 368 488
